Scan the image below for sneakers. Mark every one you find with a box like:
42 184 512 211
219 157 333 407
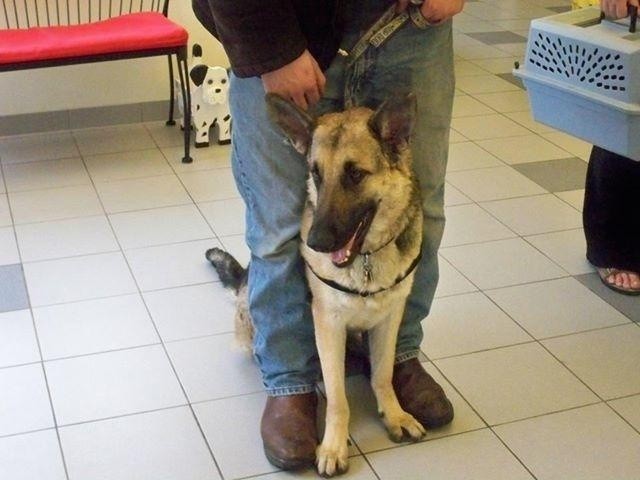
360 357 454 429
261 391 319 471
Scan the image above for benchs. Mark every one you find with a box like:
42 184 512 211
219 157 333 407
2 0 192 163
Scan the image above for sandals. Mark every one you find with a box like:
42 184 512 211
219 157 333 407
597 268 640 296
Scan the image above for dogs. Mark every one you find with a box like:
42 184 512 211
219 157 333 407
205 89 429 479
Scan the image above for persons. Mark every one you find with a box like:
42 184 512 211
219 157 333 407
192 0 466 470
582 0 640 297
